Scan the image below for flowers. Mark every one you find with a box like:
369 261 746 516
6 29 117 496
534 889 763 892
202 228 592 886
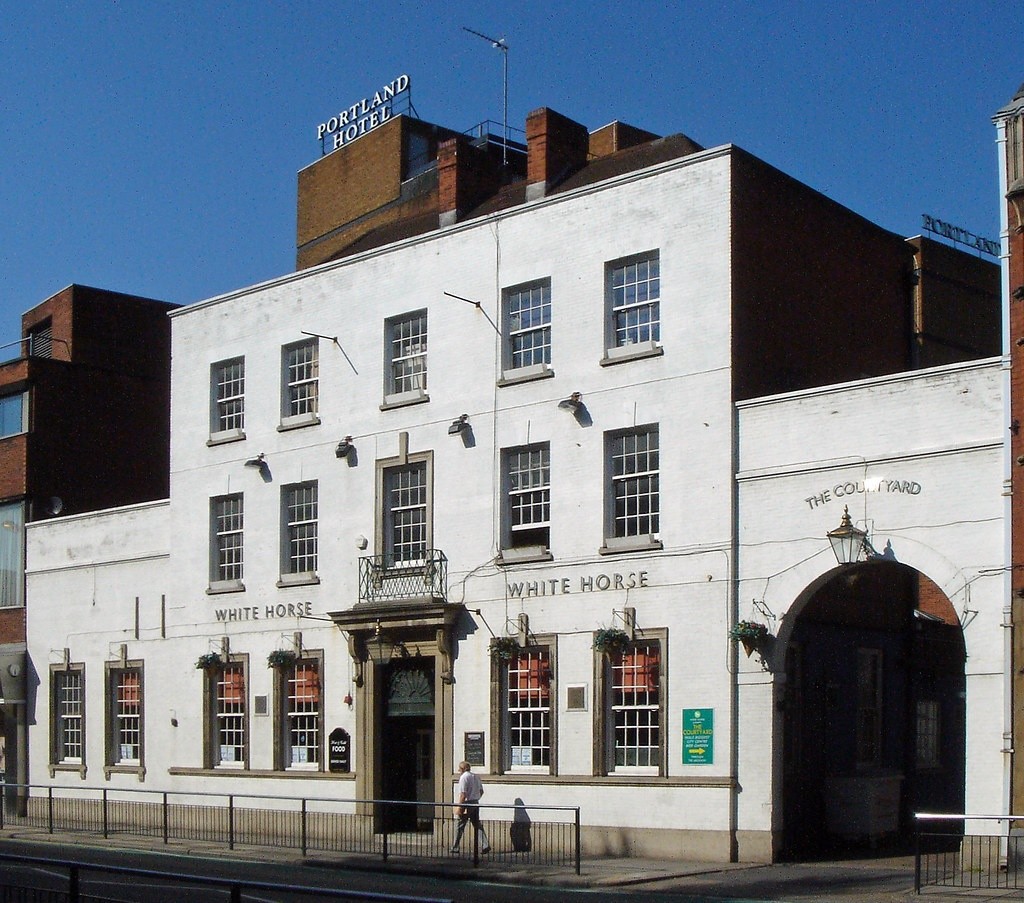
727 619 768 641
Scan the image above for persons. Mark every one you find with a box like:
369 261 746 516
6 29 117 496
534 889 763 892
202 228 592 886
450 760 491 855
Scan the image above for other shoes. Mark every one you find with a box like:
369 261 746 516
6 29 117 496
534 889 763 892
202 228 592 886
450 850 459 853
480 847 491 855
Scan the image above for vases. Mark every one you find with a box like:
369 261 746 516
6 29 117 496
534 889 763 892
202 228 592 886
740 634 759 657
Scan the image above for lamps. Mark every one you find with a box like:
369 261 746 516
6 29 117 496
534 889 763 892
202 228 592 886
558 392 582 415
827 504 867 563
448 414 470 436
244 454 264 470
365 617 398 665
335 435 353 459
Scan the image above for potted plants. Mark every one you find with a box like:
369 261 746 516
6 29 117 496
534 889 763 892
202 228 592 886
193 651 228 678
266 650 295 677
590 627 630 664
487 636 519 670
